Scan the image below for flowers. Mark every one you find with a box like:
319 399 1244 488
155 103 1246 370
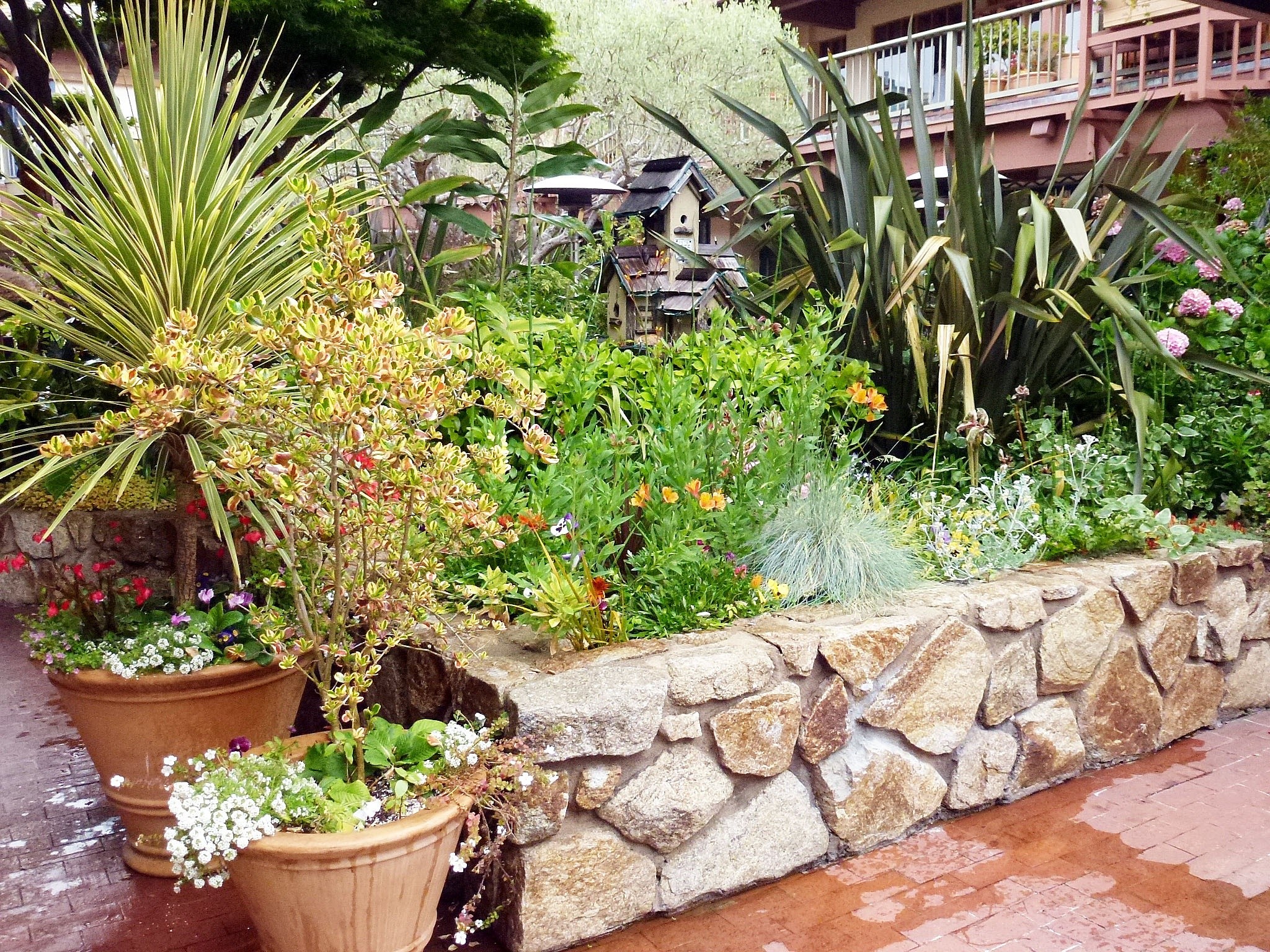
0 179 561 952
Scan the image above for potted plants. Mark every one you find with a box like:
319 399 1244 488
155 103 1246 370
973 19 1069 94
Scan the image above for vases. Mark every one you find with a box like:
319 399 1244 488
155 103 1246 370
222 732 485 952
33 634 323 875
